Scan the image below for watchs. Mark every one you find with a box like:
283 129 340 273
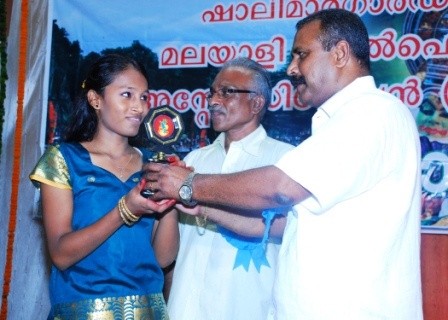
178 171 200 207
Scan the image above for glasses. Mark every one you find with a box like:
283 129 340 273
205 88 256 102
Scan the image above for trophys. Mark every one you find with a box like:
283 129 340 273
140 104 184 198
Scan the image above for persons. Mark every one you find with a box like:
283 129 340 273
29 52 180 320
143 8 422 320
166 57 296 320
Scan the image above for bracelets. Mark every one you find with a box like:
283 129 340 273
195 206 208 227
119 195 141 226
